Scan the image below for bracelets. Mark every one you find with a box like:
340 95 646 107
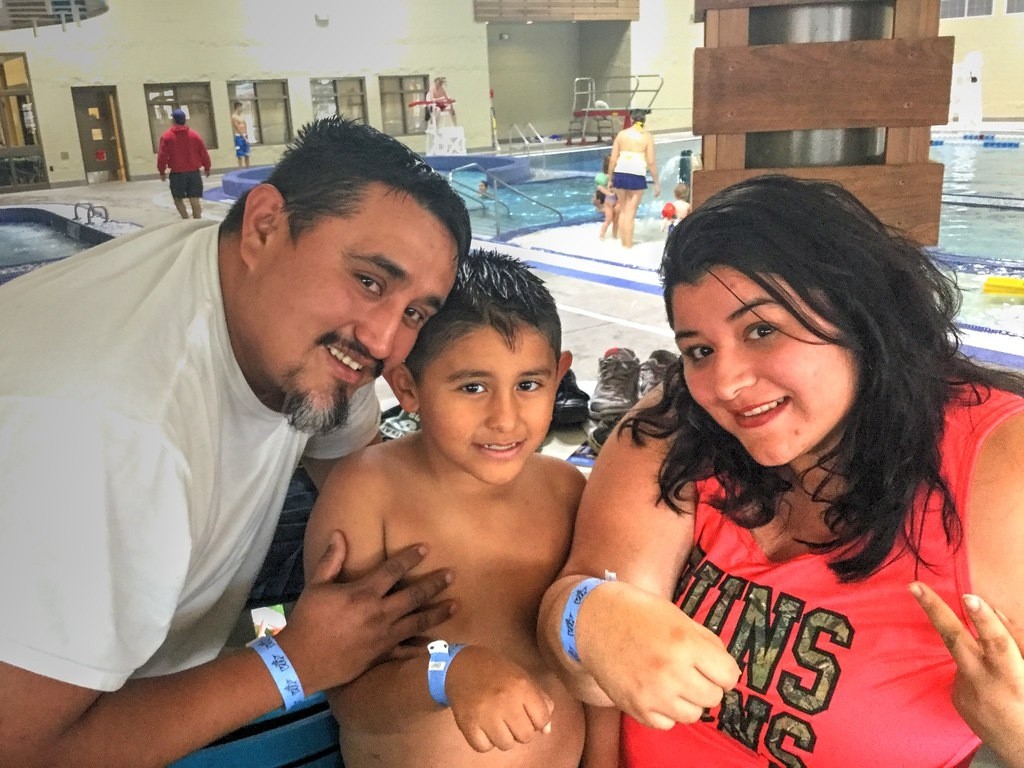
560 569 616 664
426 640 470 707
245 629 306 713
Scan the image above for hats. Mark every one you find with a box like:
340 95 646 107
171 109 186 119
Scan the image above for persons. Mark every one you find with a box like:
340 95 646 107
596 153 620 241
606 108 661 249
661 184 693 237
230 102 251 169
156 107 212 219
0 110 473 768
303 248 590 768
428 77 457 128
477 179 493 199
536 172 1024 768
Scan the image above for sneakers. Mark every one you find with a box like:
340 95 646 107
553 368 590 425
587 347 642 420
641 348 679 397
